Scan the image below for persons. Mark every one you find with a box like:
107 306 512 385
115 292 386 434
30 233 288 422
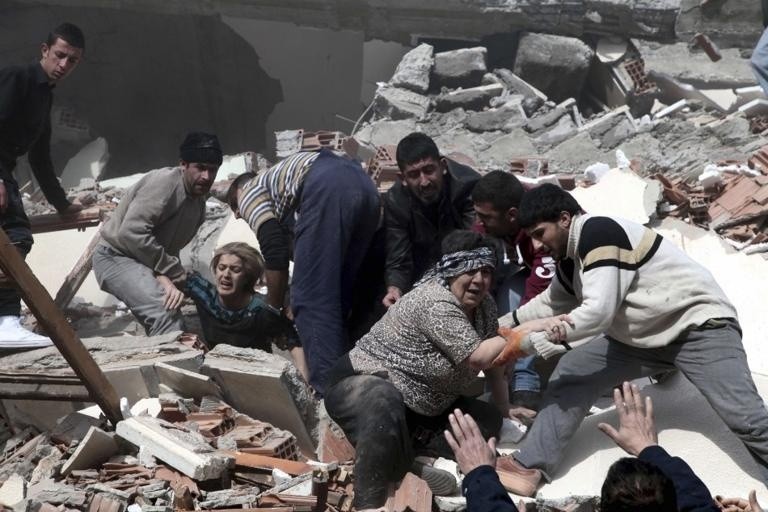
700 1 768 103
443 379 721 511
466 169 559 407
156 241 310 384
322 227 575 512
225 147 388 398
382 129 483 311
1 21 87 316
495 181 767 498
91 130 223 337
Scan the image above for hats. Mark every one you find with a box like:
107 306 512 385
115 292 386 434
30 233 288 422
180 131 223 150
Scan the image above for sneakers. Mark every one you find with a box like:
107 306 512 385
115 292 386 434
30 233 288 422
495 453 542 497
410 460 456 496
